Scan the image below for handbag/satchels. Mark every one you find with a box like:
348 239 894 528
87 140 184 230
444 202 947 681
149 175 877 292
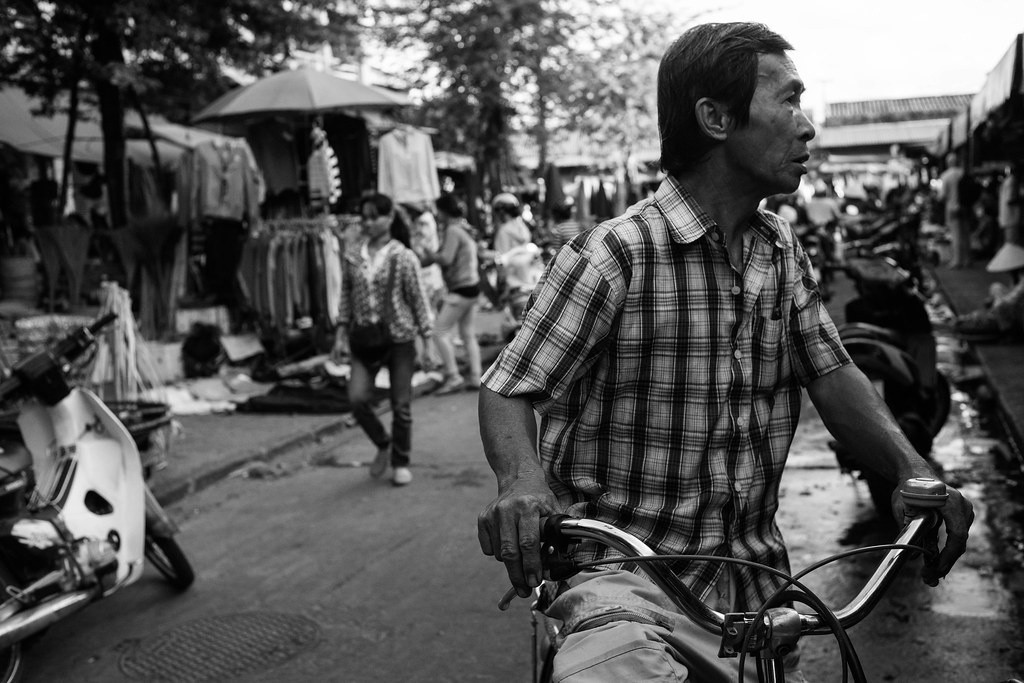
344 318 392 363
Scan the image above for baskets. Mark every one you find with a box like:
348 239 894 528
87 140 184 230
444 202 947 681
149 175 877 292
102 399 173 473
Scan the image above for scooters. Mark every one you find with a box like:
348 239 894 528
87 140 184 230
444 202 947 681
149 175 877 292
477 233 557 345
796 158 954 521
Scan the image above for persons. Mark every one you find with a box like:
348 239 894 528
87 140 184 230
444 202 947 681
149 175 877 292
759 150 1024 342
332 194 437 484
410 196 587 395
2 240 41 300
479 22 974 683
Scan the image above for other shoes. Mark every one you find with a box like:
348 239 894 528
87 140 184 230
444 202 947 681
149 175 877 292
368 445 391 479
462 375 485 391
435 379 465 396
392 468 412 484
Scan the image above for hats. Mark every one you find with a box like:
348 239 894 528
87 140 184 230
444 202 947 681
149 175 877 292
491 192 520 208
985 241 1024 271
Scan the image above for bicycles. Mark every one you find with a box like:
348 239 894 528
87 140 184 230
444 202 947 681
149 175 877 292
473 461 951 682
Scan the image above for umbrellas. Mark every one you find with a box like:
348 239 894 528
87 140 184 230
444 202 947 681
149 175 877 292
190 65 414 138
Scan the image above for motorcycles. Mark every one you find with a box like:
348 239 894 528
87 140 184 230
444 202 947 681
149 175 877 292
0 305 198 683
